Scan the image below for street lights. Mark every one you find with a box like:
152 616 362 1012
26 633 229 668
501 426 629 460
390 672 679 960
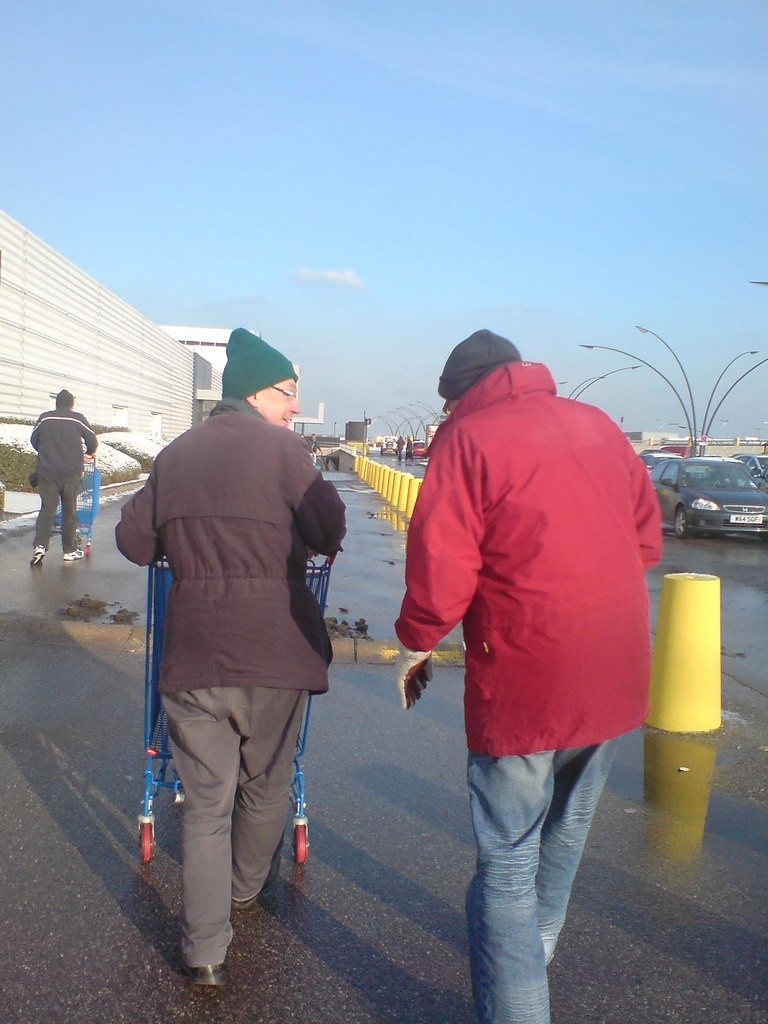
334 422 337 438
378 401 443 441
566 325 767 455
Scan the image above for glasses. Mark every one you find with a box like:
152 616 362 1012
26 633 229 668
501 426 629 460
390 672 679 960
269 385 298 403
441 399 452 417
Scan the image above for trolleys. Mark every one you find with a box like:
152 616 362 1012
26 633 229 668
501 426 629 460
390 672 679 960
138 551 336 867
33 452 102 560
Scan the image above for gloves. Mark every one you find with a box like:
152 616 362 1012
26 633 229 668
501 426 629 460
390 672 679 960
397 642 434 709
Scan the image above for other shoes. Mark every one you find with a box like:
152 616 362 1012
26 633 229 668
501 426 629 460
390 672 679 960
181 959 225 990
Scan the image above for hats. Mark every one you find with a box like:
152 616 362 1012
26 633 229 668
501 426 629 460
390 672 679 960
438 328 522 399
222 328 296 399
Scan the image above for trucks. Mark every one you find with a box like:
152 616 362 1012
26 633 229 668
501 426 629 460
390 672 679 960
426 424 439 444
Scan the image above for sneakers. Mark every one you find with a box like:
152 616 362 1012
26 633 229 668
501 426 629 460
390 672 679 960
30 545 45 567
63 549 84 560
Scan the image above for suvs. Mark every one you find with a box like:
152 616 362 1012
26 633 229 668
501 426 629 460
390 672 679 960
380 435 400 456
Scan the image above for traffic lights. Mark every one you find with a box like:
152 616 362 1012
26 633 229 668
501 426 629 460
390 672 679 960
366 419 371 425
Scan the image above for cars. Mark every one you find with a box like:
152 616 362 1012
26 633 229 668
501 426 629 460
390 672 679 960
406 440 429 459
638 451 768 546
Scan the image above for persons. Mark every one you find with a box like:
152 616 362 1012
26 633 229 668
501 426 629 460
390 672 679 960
394 330 663 1024
31 389 98 568
301 433 323 467
114 329 347 987
395 435 414 462
